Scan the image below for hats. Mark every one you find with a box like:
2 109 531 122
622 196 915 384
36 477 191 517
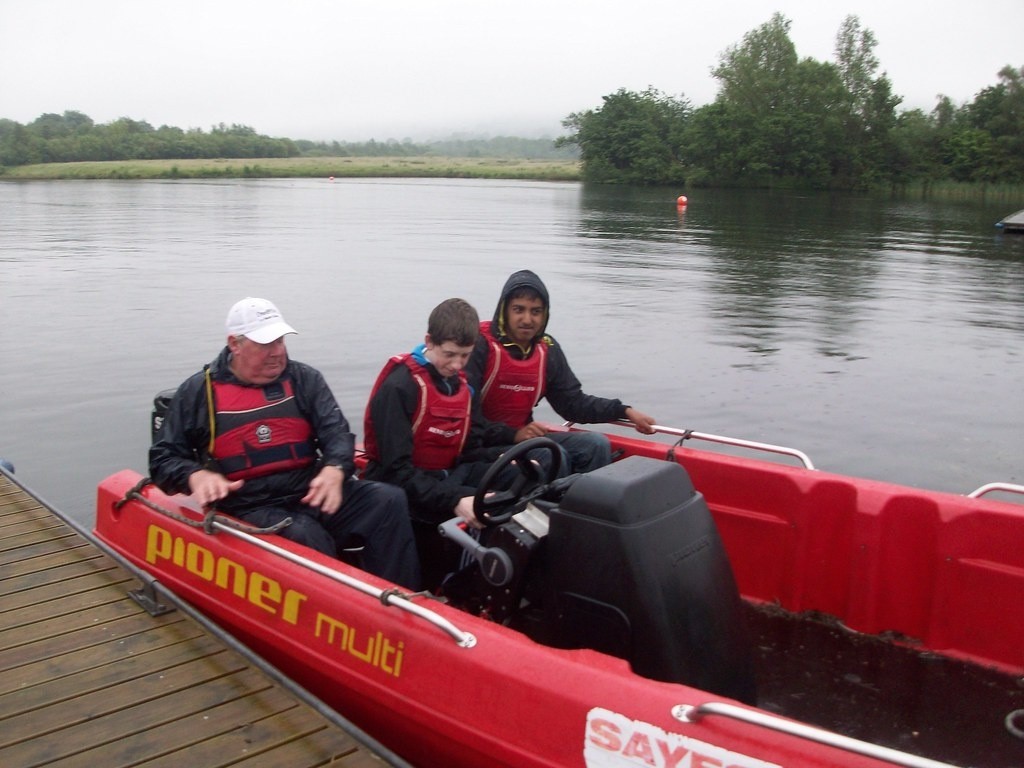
227 297 298 344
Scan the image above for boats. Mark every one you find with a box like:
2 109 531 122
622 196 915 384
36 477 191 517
90 418 1024 768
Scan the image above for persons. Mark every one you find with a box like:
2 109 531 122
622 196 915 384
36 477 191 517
360 298 548 597
462 270 658 479
150 297 426 594
